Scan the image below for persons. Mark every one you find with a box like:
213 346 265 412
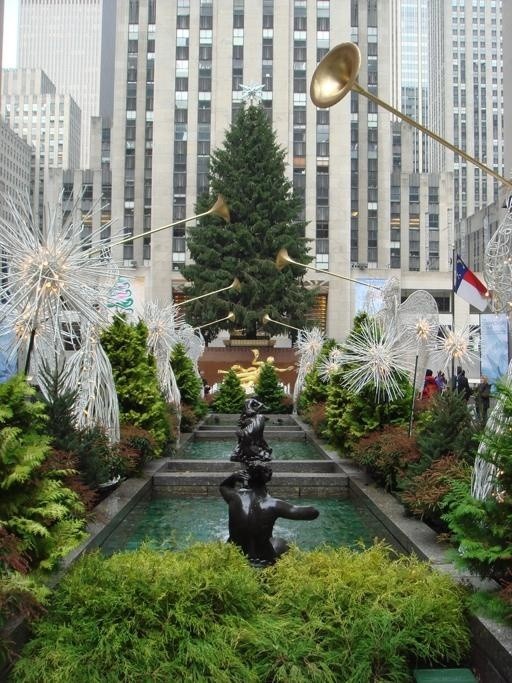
474 376 492 426
422 369 439 398
456 371 474 402
230 348 295 386
434 368 448 392
217 459 318 558
200 369 211 394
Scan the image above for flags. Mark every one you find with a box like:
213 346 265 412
454 253 490 312
